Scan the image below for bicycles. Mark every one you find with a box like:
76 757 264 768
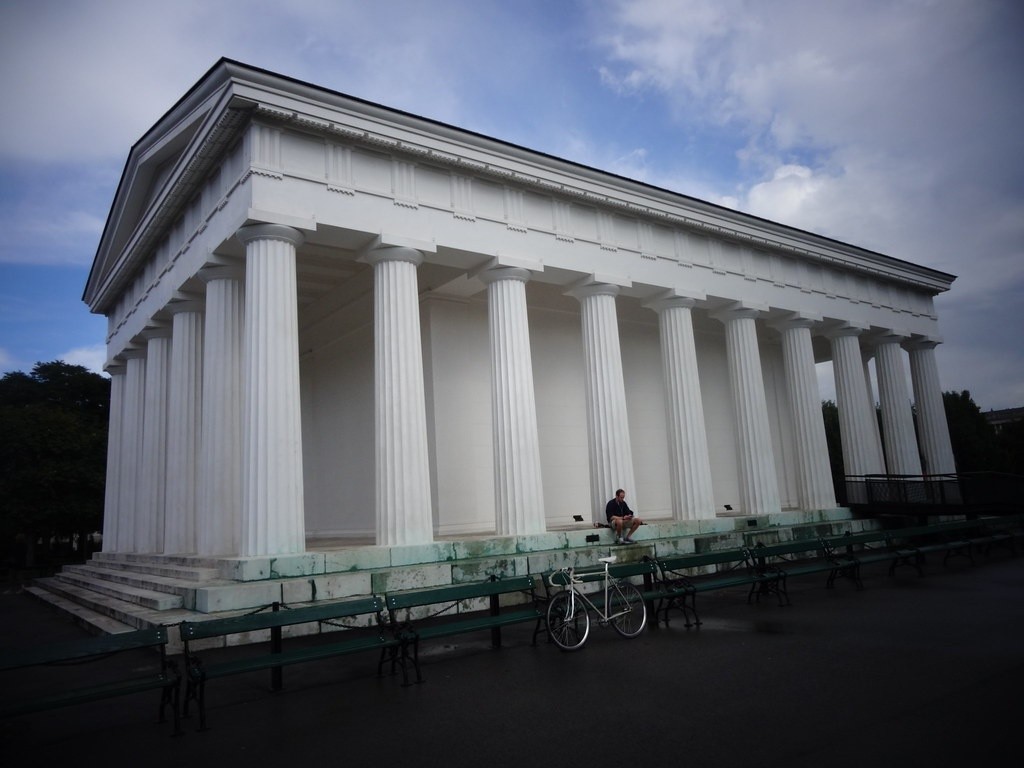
545 555 649 652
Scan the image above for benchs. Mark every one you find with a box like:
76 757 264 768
542 555 694 646
386 574 567 685
747 513 1024 606
0 626 186 737
181 595 414 733
654 547 784 627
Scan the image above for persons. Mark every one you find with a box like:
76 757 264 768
3 528 102 595
606 489 641 545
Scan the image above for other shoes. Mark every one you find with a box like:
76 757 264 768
616 535 625 544
624 537 637 544
